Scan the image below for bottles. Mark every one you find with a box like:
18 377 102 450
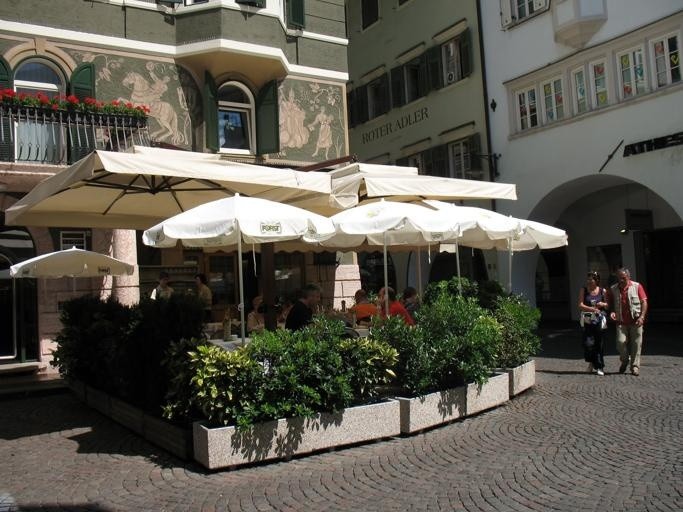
223 309 232 341
342 300 346 311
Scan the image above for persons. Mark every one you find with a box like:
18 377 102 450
144 69 170 113
607 266 647 375
305 105 332 161
278 88 309 149
320 114 334 125
576 270 611 375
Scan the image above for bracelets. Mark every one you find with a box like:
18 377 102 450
640 316 644 321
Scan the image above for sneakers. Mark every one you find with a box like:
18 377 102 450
592 367 604 375
630 367 639 376
620 360 628 372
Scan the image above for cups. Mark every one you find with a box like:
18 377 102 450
591 300 597 310
230 334 238 341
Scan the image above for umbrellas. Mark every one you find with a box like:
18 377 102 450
293 153 520 219
10 244 135 297
1 140 332 233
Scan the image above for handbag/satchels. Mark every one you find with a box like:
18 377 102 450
591 312 607 329
